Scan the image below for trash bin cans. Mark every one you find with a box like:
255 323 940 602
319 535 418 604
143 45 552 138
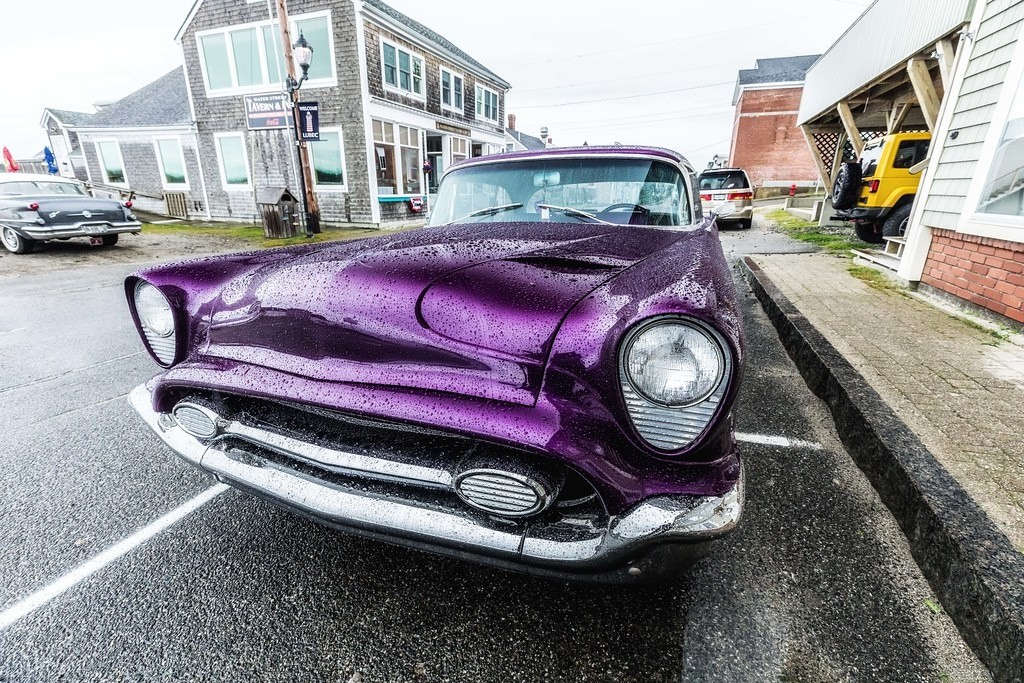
257 186 299 238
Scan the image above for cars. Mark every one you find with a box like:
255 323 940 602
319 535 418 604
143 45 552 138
125 146 748 586
0 172 141 256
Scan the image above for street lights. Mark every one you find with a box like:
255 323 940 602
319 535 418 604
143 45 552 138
284 28 314 238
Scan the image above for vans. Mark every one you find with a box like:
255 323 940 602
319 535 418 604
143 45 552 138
696 167 756 229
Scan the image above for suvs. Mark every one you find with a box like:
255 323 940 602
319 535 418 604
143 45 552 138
829 130 932 245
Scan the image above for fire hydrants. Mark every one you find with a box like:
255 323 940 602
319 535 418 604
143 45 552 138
790 183 797 196
